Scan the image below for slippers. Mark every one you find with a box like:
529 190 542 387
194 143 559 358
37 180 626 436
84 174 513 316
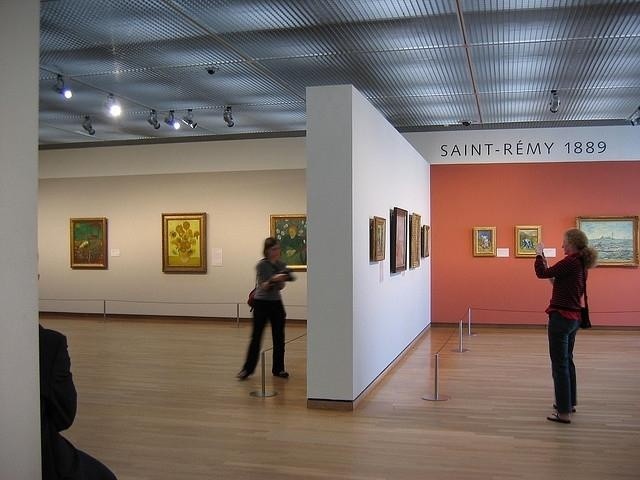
552 402 577 413
547 411 572 425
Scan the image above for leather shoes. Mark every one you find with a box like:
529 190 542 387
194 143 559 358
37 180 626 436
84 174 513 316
272 370 289 378
235 368 254 377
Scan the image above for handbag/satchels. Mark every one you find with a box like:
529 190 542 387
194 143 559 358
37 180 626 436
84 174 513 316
247 288 258 312
581 266 592 330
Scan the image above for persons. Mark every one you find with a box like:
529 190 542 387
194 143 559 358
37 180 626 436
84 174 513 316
38 320 118 480
522 238 532 249
480 235 489 249
235 236 297 378
534 227 599 425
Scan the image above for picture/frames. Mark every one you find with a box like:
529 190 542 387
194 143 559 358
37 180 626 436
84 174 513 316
69 216 108 270
576 215 640 267
514 225 542 258
161 212 207 273
371 207 430 273
473 226 497 257
269 213 307 272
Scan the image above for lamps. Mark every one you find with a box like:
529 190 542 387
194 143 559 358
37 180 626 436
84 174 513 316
631 115 640 126
550 95 561 113
51 76 234 136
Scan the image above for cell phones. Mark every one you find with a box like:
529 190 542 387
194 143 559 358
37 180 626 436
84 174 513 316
542 249 546 258
